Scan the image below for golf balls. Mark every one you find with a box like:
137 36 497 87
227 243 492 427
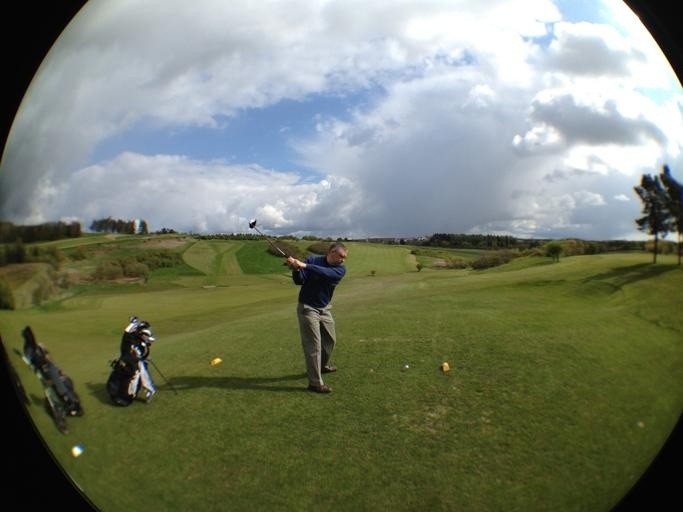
405 364 409 369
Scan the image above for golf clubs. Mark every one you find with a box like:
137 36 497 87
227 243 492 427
129 317 155 348
248 219 301 270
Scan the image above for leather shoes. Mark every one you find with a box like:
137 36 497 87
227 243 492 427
321 367 336 373
307 384 332 392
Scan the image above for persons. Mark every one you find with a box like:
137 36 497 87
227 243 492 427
286 243 349 393
